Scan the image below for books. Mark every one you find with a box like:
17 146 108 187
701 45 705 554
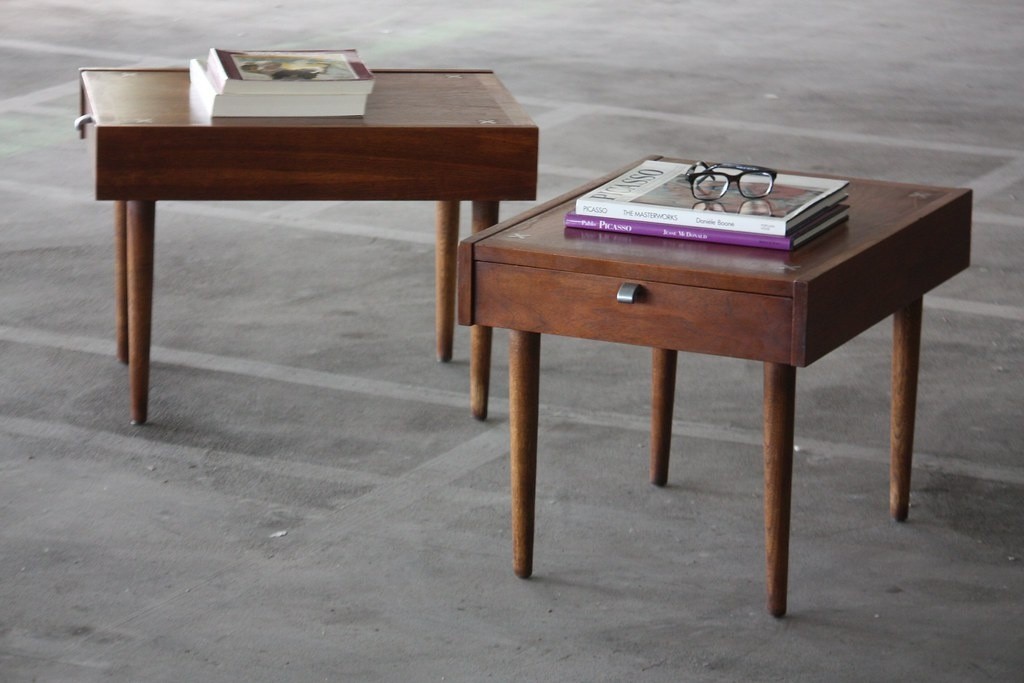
190 48 375 118
564 159 852 251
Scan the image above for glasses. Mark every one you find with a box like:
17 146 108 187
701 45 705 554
685 161 777 201
692 200 773 218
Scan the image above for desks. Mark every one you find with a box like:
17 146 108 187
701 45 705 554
458 155 973 617
78 67 542 422
